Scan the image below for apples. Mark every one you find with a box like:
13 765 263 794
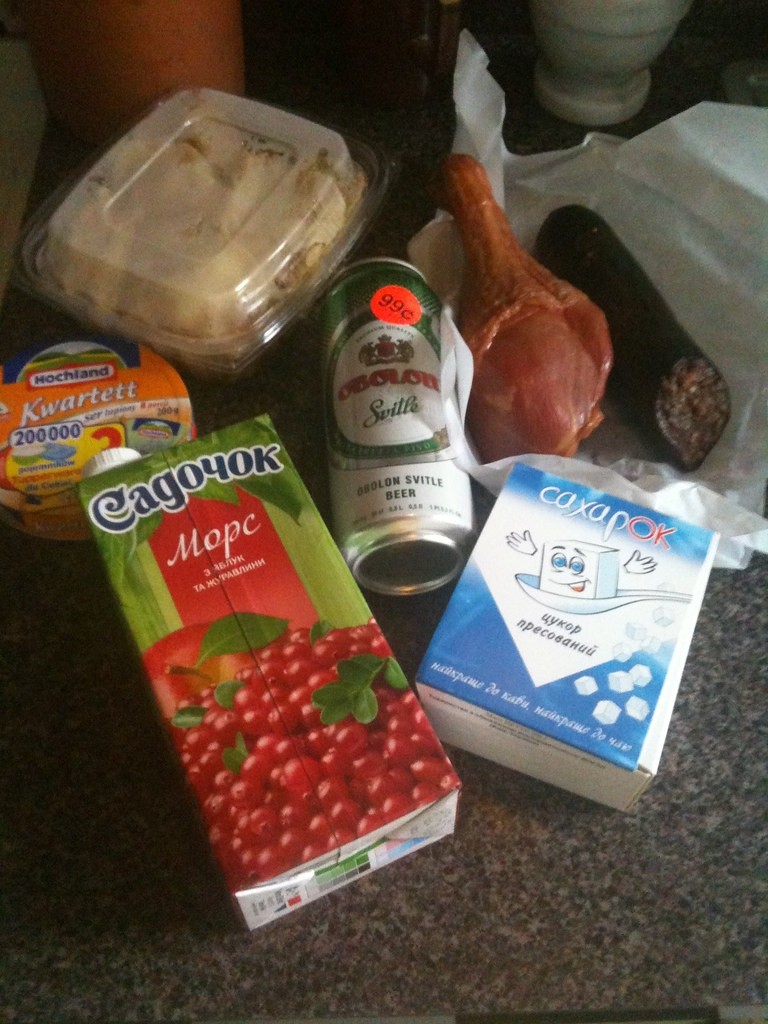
139 610 288 728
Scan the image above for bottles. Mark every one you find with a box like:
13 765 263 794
526 1 697 130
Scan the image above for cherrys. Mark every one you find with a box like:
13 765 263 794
177 622 462 895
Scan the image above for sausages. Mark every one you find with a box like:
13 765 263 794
539 206 730 474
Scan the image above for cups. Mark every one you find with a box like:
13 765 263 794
21 0 245 150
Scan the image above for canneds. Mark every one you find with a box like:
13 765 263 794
318 258 477 595
